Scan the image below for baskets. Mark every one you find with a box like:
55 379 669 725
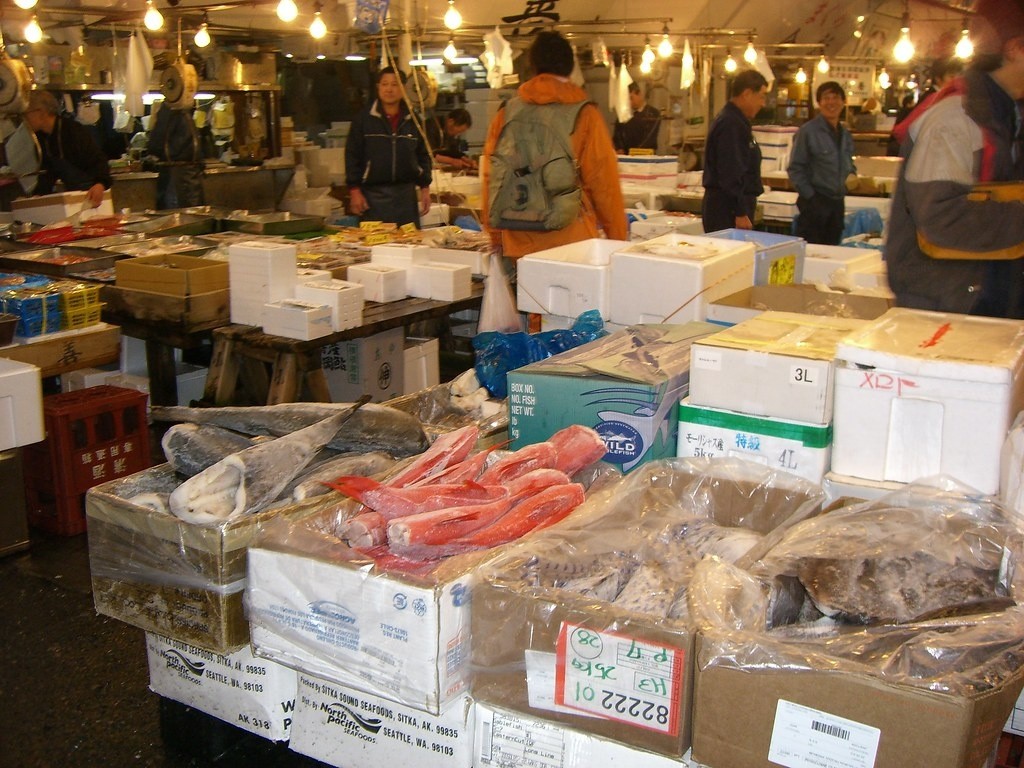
3 280 108 338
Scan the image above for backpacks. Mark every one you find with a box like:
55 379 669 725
486 97 597 231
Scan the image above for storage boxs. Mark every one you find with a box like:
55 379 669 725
0 130 1024 767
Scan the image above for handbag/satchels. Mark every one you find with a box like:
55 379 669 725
477 253 522 334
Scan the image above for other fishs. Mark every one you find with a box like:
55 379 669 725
130 384 607 572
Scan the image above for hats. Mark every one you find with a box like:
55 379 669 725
30 90 61 115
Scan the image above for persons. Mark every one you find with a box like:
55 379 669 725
17 88 114 208
482 26 630 335
702 70 769 233
887 0 1024 320
145 98 206 210
342 67 432 229
787 81 859 245
613 82 661 154
421 107 479 169
